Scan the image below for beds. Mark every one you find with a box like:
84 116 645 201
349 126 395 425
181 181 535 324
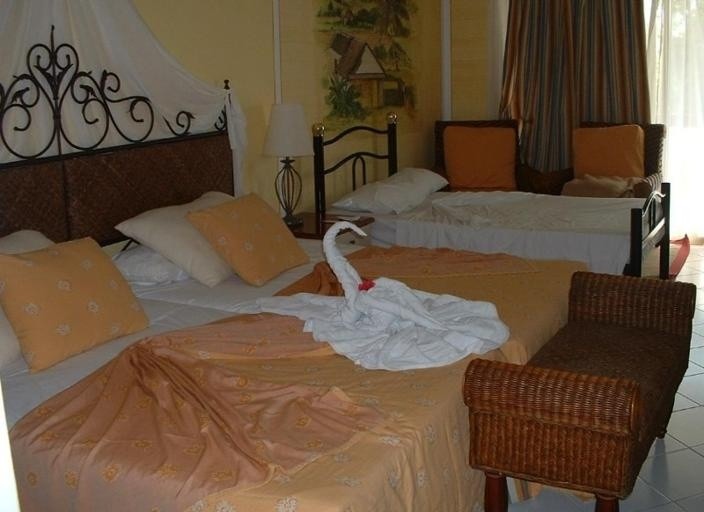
312 112 670 280
1 25 588 511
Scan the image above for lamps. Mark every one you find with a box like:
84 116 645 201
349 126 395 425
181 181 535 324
262 103 314 226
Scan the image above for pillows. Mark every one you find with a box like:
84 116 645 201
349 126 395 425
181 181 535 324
0 238 150 375
442 126 516 188
113 191 237 286
119 245 193 285
187 192 309 286
0 229 56 366
570 124 645 178
331 166 449 214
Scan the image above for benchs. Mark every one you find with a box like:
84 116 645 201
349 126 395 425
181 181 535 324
464 270 696 511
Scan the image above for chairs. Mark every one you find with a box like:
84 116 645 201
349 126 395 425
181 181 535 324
433 120 518 192
548 122 666 198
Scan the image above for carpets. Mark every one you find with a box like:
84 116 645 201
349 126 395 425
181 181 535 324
641 233 691 280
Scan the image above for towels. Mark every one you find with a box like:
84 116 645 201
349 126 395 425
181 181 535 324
256 221 510 372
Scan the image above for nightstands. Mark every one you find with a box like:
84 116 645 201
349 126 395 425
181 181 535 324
285 213 375 245
